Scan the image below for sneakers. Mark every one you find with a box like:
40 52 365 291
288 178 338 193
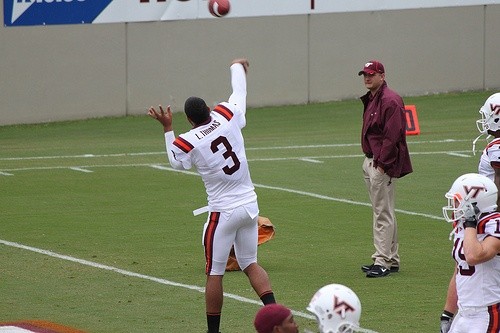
361 263 399 278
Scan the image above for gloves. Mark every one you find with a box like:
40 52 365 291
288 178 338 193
459 202 479 228
440 310 455 333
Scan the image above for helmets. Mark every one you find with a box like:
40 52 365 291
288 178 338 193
441 173 497 224
478 93 500 132
306 283 361 333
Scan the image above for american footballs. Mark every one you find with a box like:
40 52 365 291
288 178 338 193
209 0 230 17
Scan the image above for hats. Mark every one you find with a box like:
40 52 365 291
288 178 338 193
358 61 385 76
254 304 291 333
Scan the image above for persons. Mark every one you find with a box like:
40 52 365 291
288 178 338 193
254 305 299 333
145 58 277 333
477 92 500 210
440 174 500 333
356 61 403 278
